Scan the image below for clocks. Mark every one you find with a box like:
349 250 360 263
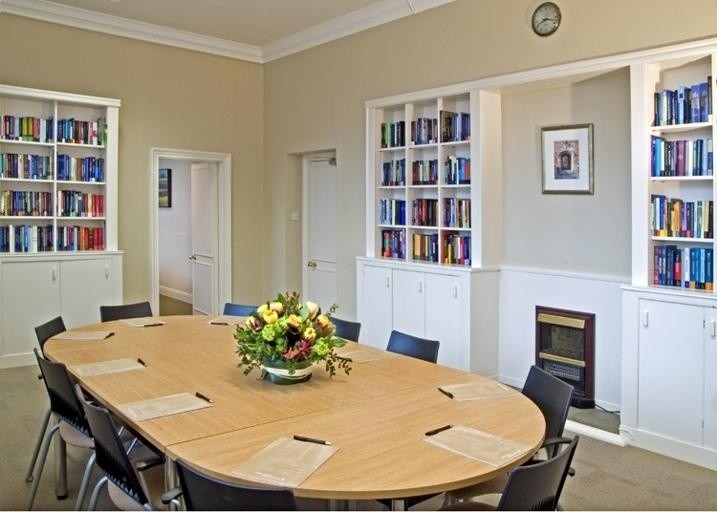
531 2 561 35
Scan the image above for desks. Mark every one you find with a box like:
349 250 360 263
54 314 546 511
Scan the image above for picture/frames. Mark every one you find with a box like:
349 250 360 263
539 121 594 196
159 168 171 208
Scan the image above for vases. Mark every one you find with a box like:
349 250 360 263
262 357 313 384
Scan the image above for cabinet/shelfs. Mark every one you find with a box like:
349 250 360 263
0 82 123 368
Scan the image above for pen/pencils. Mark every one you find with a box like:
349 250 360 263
196 392 213 402
211 322 228 325
336 356 352 362
426 424 454 435
437 387 453 398
294 435 333 445
144 324 163 327
104 333 114 339
137 358 148 367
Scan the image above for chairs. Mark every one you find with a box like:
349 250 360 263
386 330 439 363
100 301 152 321
173 458 299 510
27 348 135 511
522 366 576 456
224 303 258 316
441 433 579 510
25 316 66 483
73 384 169 512
330 316 361 343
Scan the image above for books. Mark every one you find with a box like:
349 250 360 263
381 110 470 264
650 75 713 290
0 115 106 252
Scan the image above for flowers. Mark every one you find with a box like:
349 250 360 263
234 291 352 380
